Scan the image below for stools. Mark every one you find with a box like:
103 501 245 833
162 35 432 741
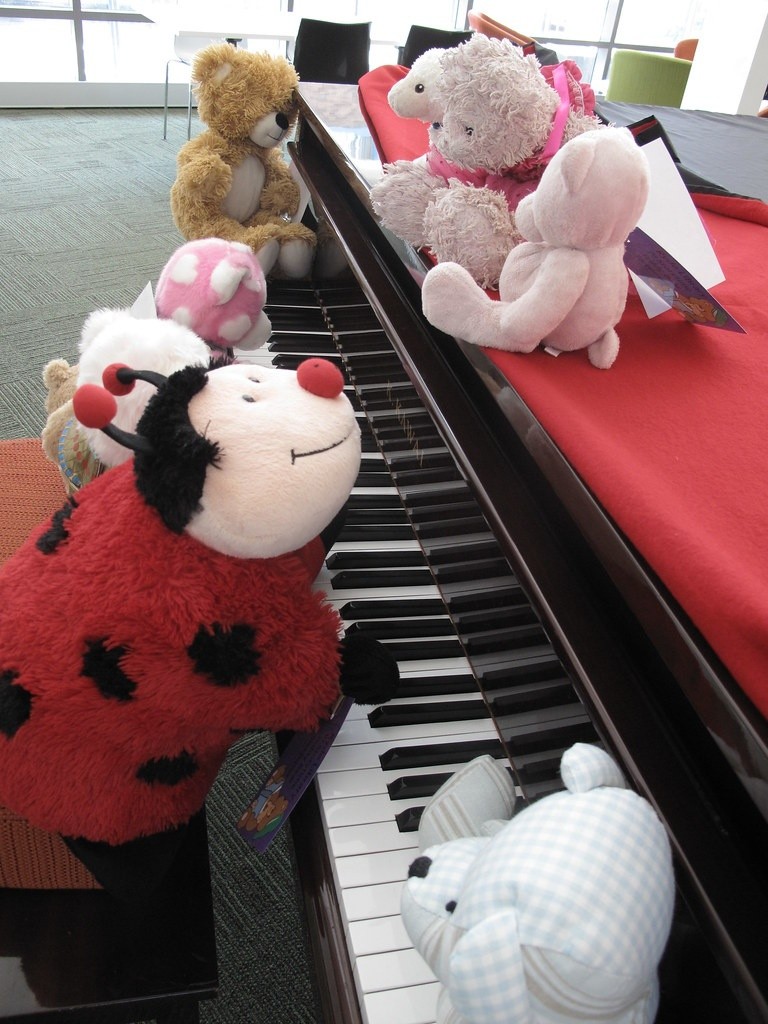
161 31 229 144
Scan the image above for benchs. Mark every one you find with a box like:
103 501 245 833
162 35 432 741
0 439 221 1023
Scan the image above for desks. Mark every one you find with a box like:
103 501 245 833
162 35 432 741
179 25 404 63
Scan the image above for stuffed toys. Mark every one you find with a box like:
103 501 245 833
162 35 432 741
172 43 318 281
370 32 649 367
400 744 676 1024
0 238 400 902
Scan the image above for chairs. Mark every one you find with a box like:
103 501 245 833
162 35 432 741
291 16 371 85
399 24 474 69
466 9 535 48
606 50 692 110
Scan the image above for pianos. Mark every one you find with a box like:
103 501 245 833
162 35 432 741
224 80 768 1024
